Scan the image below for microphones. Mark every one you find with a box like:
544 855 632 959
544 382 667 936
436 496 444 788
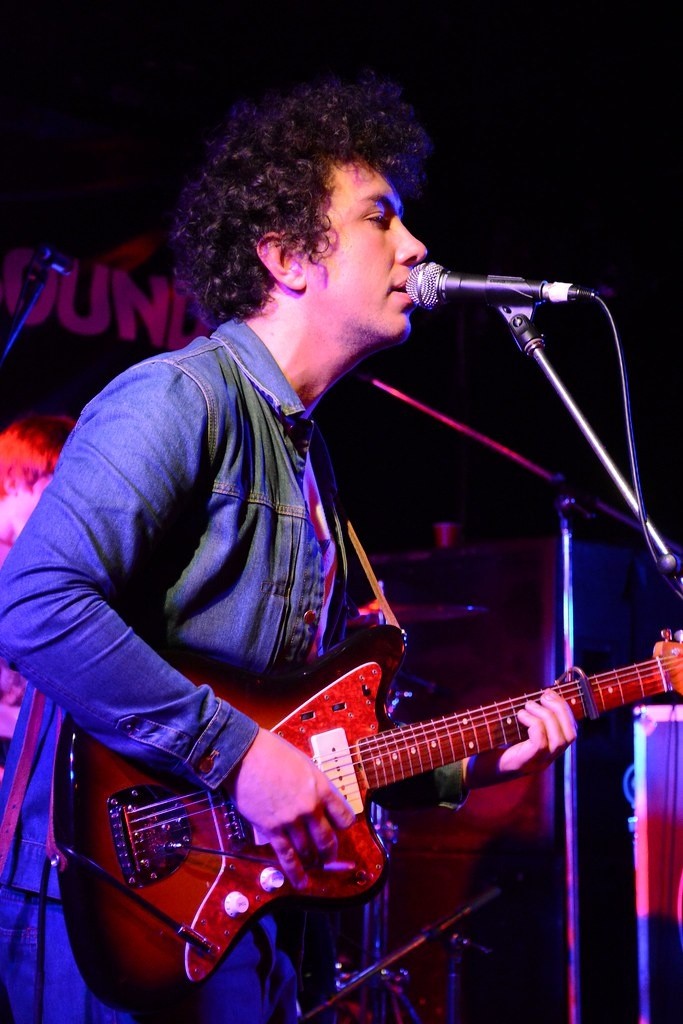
406 262 600 309
40 247 74 275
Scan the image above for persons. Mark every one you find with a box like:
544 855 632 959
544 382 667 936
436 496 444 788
0 76 580 1023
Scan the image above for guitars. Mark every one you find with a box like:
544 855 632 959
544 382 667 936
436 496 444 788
50 625 681 1018
